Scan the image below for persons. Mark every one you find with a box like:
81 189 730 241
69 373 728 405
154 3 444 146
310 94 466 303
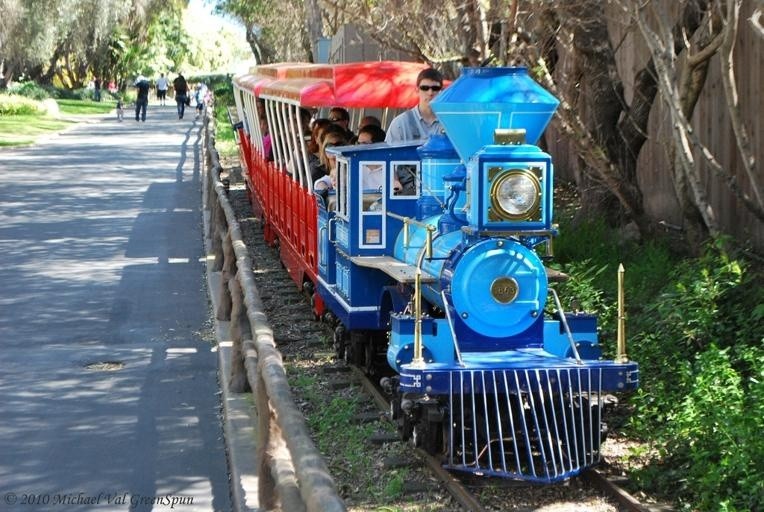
254 97 386 192
382 67 445 195
92 71 208 122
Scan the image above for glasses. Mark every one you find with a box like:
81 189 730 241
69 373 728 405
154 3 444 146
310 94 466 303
330 117 342 121
419 85 442 91
325 142 344 147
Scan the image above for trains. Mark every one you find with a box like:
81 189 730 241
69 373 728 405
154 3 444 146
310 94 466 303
222 49 643 486
